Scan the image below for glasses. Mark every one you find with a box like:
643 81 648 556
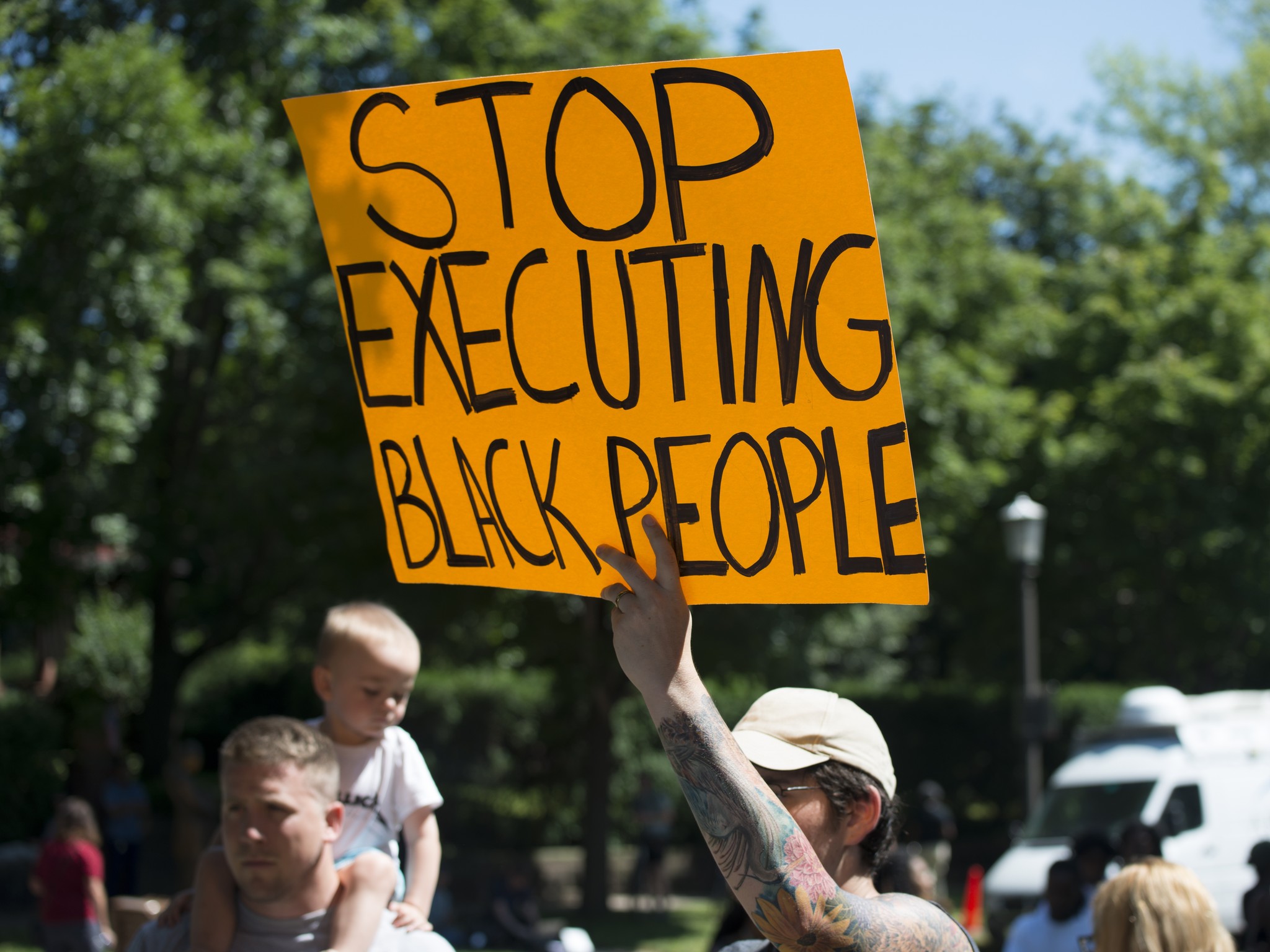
768 782 821 799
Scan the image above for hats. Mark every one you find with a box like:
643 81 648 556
731 687 896 801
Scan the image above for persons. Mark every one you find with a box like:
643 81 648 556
26 797 119 951
999 819 1270 951
130 595 454 952
878 779 962 904
587 512 982 952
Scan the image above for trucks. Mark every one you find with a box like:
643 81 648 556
966 679 1270 952
